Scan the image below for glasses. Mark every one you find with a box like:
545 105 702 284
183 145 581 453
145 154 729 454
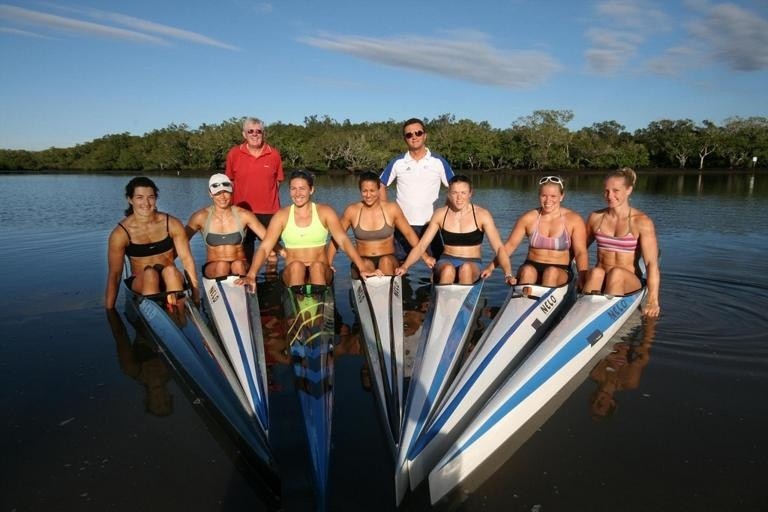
539 176 563 189
244 129 264 135
404 130 424 139
209 182 232 188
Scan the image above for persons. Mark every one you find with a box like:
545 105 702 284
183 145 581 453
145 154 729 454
173 175 288 280
327 171 436 277
235 170 386 295
380 119 458 258
226 119 285 263
105 312 173 418
588 318 657 422
105 177 203 311
394 176 516 286
257 303 499 398
479 176 590 290
570 167 661 317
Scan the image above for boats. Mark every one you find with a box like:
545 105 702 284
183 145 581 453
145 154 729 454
426 261 648 511
357 273 406 462
123 272 285 509
391 270 484 508
407 279 574 491
289 267 336 512
200 266 269 437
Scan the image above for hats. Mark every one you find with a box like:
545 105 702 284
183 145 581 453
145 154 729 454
208 173 233 194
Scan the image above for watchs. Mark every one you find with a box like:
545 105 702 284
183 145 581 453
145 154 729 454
505 274 513 278
